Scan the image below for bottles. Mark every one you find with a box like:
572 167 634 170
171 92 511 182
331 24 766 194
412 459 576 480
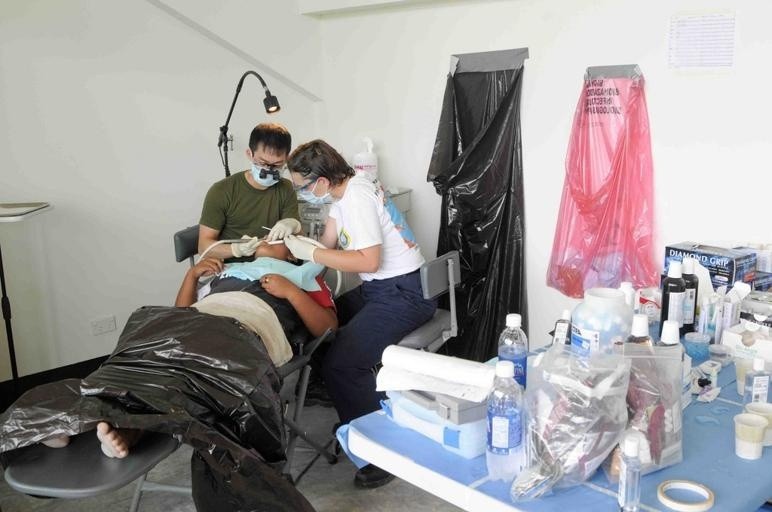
552 309 573 345
486 360 528 482
497 310 528 389
740 357 772 407
655 319 682 350
625 312 654 349
660 259 685 340
618 434 642 512
681 257 699 333
618 280 635 305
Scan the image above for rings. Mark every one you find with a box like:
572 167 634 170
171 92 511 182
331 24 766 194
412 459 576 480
264 277 269 282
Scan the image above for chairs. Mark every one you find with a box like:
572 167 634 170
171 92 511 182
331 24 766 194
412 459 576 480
370 249 464 396
174 224 200 269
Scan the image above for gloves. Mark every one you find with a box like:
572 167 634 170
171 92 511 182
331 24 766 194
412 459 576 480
232 234 262 258
266 218 301 242
284 235 318 264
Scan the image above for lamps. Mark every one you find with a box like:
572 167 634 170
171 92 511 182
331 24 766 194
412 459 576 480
216 71 284 179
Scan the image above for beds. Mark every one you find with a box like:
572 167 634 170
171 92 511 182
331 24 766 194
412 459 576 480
5 311 340 512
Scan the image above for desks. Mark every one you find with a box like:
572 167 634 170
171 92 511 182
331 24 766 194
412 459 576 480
342 292 772 512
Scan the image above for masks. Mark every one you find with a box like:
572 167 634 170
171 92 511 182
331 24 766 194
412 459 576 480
297 180 332 204
251 154 284 187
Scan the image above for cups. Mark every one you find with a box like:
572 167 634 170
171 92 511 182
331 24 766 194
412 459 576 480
732 350 767 396
699 296 743 344
684 330 710 365
731 412 769 461
744 401 770 448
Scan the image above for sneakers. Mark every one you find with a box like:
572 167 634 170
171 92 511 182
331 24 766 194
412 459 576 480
354 464 394 489
294 381 334 407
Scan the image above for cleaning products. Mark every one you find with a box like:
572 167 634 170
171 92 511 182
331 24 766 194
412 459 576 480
352 138 378 180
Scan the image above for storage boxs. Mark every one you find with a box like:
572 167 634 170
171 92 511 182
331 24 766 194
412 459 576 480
659 269 771 293
661 241 757 288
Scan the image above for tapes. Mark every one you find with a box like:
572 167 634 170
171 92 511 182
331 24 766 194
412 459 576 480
658 480 714 512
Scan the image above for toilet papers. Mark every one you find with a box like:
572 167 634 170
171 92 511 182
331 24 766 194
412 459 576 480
375 344 497 403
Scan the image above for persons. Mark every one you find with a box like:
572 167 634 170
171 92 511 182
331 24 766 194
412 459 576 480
196 123 338 409
287 138 438 489
39 233 339 459
